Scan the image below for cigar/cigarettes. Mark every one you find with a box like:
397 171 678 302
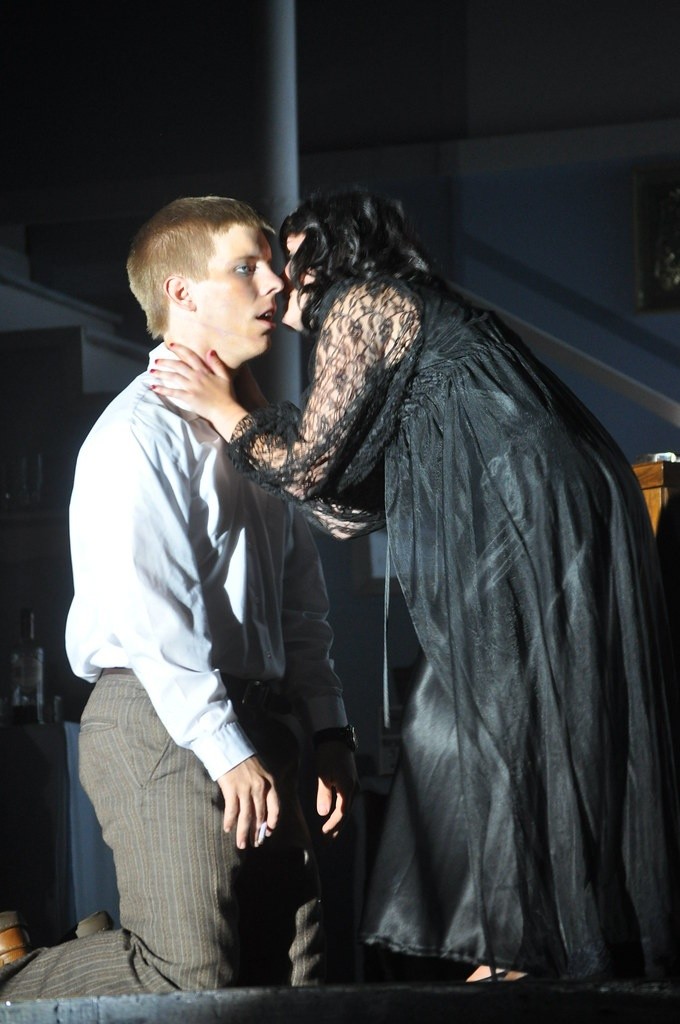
259 822 268 846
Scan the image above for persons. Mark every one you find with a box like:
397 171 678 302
148 190 679 981
0 196 365 1003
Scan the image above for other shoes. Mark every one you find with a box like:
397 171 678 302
0 910 33 968
61 910 115 942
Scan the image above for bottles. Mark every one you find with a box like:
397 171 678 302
9 610 45 725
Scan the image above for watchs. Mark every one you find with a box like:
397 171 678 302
312 723 360 753
